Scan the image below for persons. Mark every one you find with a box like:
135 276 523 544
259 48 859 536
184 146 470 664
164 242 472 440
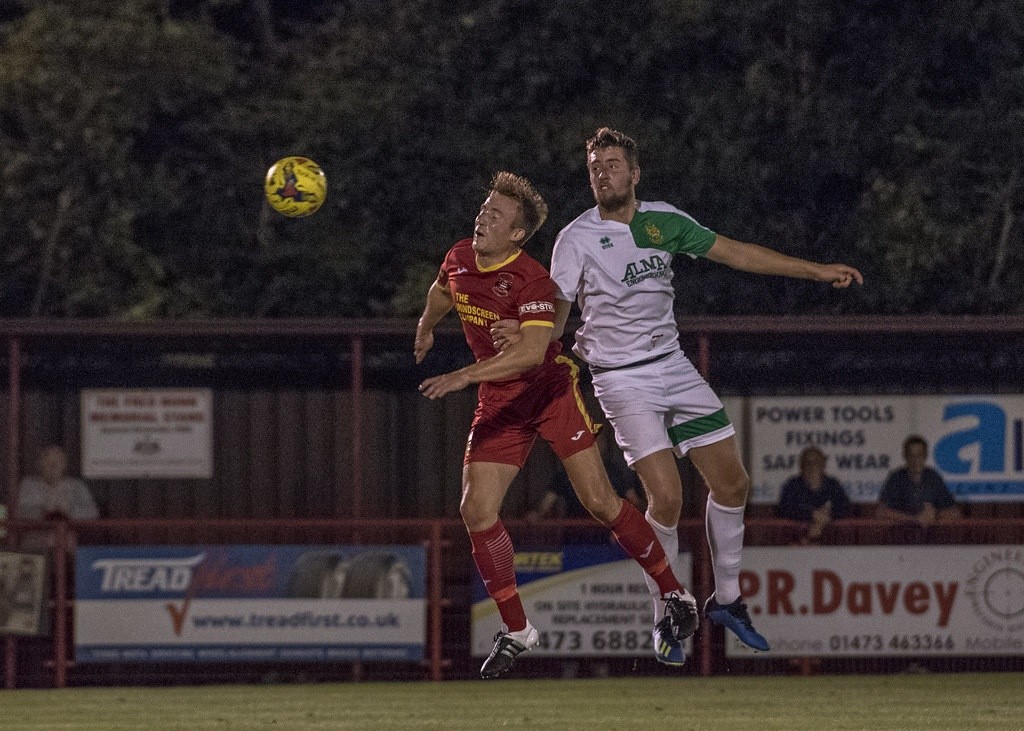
18 440 100 688
414 170 699 679
523 431 958 674
487 127 864 667
1 0 1024 388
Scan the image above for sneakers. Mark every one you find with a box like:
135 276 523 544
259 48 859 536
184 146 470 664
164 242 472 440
660 587 699 641
480 618 539 679
703 591 770 654
652 616 686 666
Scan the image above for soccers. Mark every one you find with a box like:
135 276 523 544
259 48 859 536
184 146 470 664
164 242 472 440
265 156 327 219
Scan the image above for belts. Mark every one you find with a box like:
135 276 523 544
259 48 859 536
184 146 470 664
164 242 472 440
590 350 675 370
